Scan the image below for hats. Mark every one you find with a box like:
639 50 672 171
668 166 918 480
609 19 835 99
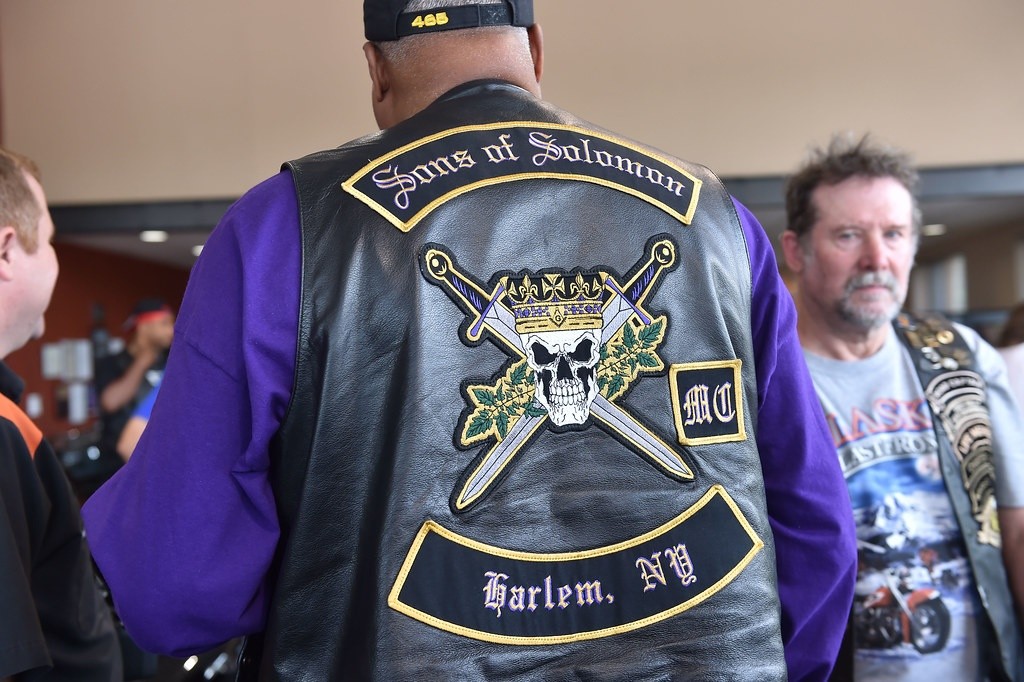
363 0 534 42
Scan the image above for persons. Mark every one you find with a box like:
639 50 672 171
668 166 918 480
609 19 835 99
779 132 1023 682
91 297 175 493
78 0 857 682
0 148 133 682
